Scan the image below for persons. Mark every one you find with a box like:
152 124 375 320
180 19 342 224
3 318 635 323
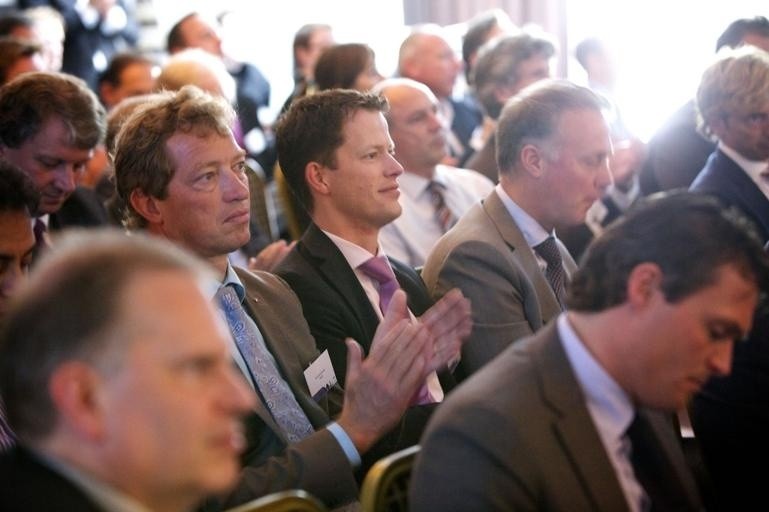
1 0 769 512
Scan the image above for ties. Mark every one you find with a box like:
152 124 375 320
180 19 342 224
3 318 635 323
30 219 49 269
427 180 458 233
533 235 573 314
358 255 437 405
216 284 315 446
